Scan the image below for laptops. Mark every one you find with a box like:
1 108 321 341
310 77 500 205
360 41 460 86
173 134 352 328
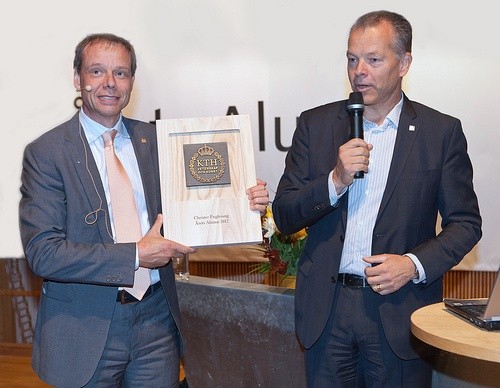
444 267 500 331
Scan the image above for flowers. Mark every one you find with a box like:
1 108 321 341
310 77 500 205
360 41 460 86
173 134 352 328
245 205 308 283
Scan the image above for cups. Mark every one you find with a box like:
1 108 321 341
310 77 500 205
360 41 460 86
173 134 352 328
171 253 189 281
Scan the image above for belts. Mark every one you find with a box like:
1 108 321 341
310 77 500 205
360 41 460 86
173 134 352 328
337 272 370 288
116 280 162 304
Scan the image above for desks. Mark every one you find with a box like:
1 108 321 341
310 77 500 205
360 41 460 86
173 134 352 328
410 298 500 363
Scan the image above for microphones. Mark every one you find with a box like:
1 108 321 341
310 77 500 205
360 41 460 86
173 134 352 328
78 85 92 91
347 92 365 178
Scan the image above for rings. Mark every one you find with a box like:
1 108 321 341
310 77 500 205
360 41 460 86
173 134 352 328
377 285 383 293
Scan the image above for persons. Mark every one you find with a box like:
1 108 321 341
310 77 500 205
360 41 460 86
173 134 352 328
273 7 485 388
16 31 271 388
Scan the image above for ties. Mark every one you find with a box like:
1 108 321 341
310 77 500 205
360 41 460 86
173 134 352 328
100 130 151 302
